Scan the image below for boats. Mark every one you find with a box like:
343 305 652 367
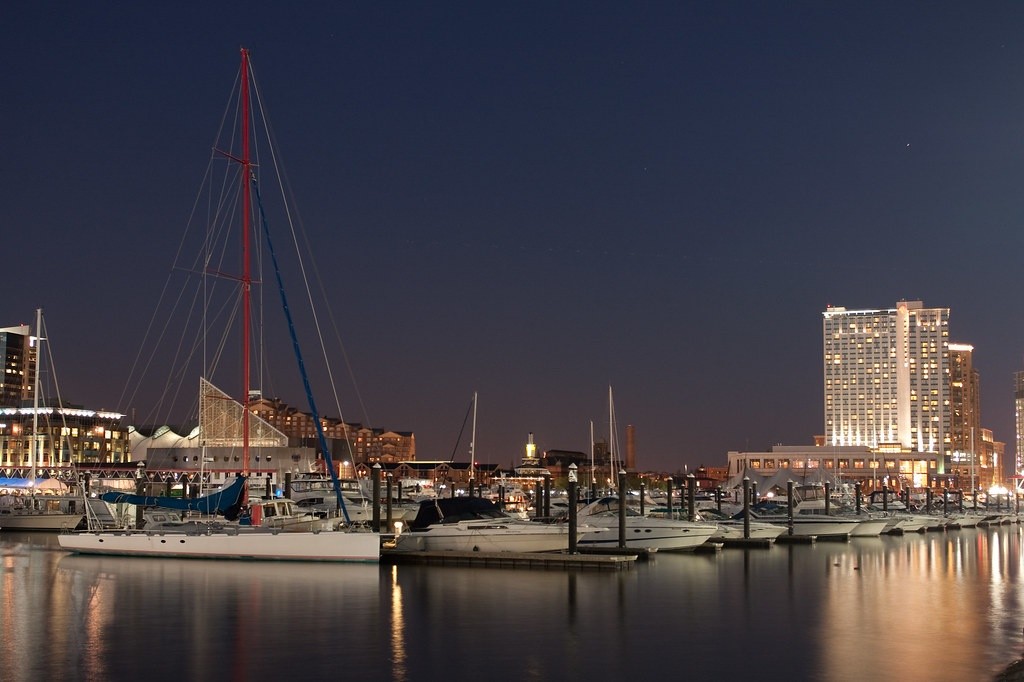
88 385 1024 559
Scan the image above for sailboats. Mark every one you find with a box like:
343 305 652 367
1 308 88 531
59 45 382 564
